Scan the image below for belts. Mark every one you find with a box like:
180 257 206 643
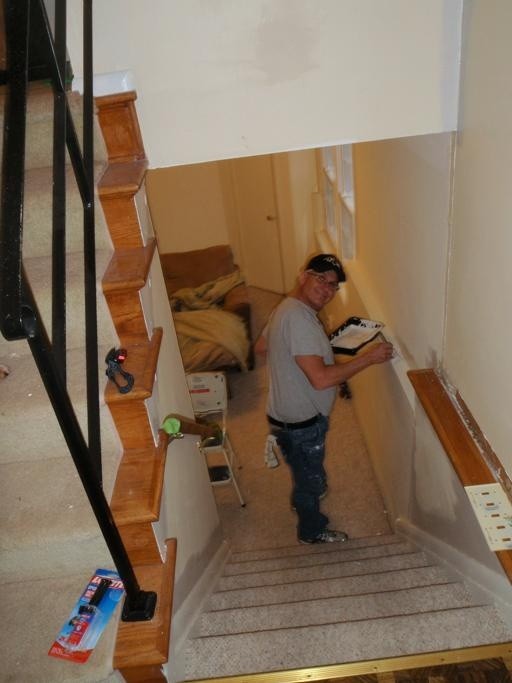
266 412 323 430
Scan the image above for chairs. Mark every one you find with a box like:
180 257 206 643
185 372 247 506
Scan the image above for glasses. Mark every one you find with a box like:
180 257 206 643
306 271 339 289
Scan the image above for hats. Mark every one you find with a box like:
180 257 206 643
308 253 345 281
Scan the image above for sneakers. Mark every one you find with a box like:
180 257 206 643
289 483 328 511
297 526 348 544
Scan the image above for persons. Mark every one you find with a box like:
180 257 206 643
267 253 393 545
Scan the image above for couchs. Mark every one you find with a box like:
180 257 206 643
159 244 254 371
